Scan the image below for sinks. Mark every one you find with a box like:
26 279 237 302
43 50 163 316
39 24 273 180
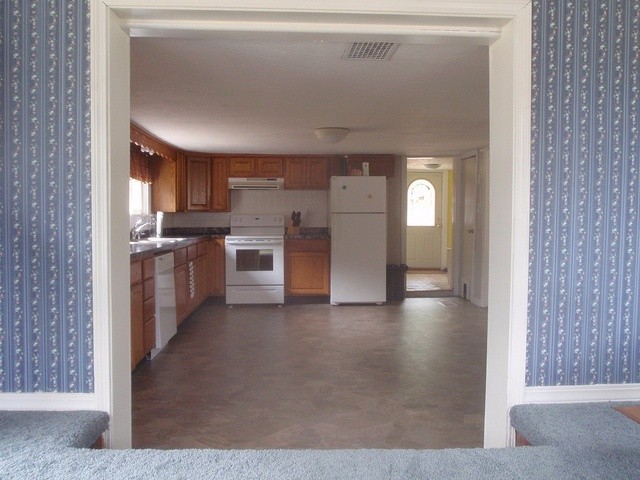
130 239 176 247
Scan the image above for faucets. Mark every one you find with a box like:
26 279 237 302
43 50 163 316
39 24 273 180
130 221 155 241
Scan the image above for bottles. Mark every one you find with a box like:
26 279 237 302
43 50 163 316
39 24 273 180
341 155 348 175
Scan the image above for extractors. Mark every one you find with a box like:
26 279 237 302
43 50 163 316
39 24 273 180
227 177 284 190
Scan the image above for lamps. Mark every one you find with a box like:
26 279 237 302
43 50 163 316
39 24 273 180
313 128 350 145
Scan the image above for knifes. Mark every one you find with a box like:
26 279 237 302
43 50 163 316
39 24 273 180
292 211 301 226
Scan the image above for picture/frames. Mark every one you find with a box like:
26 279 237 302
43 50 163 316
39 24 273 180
151 150 187 212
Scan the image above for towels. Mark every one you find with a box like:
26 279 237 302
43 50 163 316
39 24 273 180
189 262 195 299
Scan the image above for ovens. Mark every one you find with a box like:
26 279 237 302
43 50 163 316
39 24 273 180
225 239 285 308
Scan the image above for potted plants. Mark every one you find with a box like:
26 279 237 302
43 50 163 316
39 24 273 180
291 210 302 235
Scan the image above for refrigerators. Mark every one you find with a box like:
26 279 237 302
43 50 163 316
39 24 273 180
329 175 387 305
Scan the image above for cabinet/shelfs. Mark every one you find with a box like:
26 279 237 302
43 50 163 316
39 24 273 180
286 230 331 297
206 235 225 298
194 234 207 306
344 154 395 178
328 155 344 177
230 154 284 178
171 234 198 326
285 153 327 190
187 153 229 211
130 240 143 369
143 237 155 358
